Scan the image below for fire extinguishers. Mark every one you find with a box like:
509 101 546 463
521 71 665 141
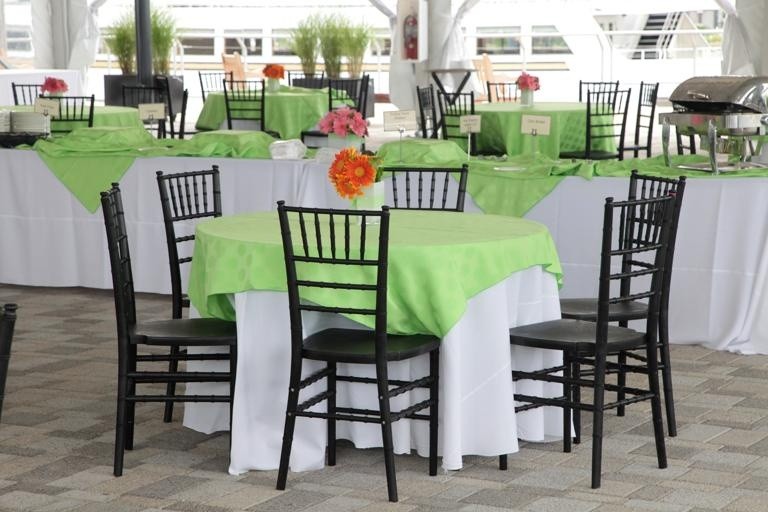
401 5 418 59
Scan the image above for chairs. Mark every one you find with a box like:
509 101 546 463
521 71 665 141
301 74 364 149
178 88 188 140
99 181 242 476
374 163 470 212
197 71 235 104
616 81 659 157
436 89 507 157
156 164 224 422
560 169 694 442
121 84 165 140
560 88 634 161
499 189 671 488
12 81 45 106
358 74 370 150
288 70 324 89
579 80 619 114
275 200 441 503
222 78 281 138
487 81 520 102
153 74 211 139
416 84 438 139
40 93 95 137
